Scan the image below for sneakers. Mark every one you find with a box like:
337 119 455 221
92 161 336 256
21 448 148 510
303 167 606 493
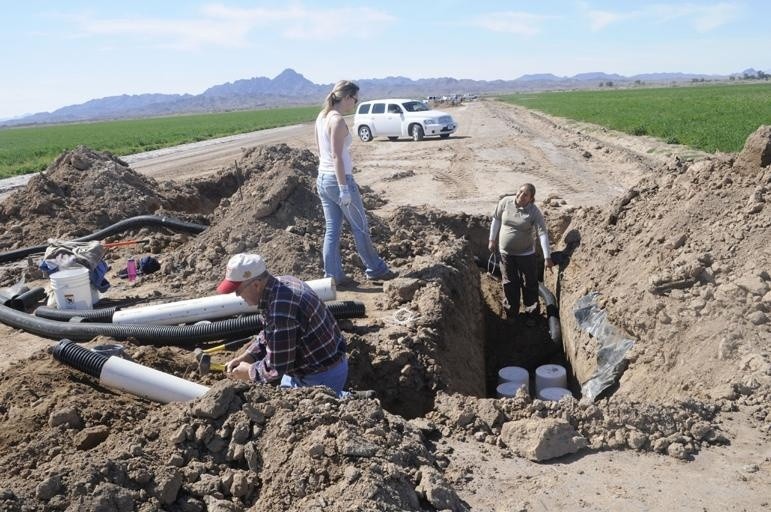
335 273 355 283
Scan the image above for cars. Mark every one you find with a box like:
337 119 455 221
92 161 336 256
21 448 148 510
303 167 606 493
423 93 479 104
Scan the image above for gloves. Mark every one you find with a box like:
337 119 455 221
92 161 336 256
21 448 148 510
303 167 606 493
338 184 353 209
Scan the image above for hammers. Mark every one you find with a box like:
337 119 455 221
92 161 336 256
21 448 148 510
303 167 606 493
193 336 253 362
199 354 236 379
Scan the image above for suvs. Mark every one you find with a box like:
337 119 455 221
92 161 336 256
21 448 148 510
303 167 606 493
351 98 459 142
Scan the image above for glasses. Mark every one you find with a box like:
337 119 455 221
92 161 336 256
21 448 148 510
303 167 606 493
346 93 359 105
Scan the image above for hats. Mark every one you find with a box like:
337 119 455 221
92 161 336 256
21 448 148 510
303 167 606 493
215 251 266 295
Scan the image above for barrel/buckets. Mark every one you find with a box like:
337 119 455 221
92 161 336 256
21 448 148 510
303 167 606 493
497 366 530 390
539 387 573 404
534 363 567 396
50 268 94 312
496 383 528 397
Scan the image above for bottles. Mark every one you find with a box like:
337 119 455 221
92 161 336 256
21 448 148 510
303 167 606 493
126 258 136 279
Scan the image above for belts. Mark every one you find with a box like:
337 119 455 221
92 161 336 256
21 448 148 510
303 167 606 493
301 354 346 375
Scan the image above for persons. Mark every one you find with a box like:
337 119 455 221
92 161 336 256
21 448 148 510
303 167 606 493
217 252 350 395
312 80 400 291
488 181 557 329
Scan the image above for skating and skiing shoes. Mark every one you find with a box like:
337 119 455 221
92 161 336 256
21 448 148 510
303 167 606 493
364 269 400 280
